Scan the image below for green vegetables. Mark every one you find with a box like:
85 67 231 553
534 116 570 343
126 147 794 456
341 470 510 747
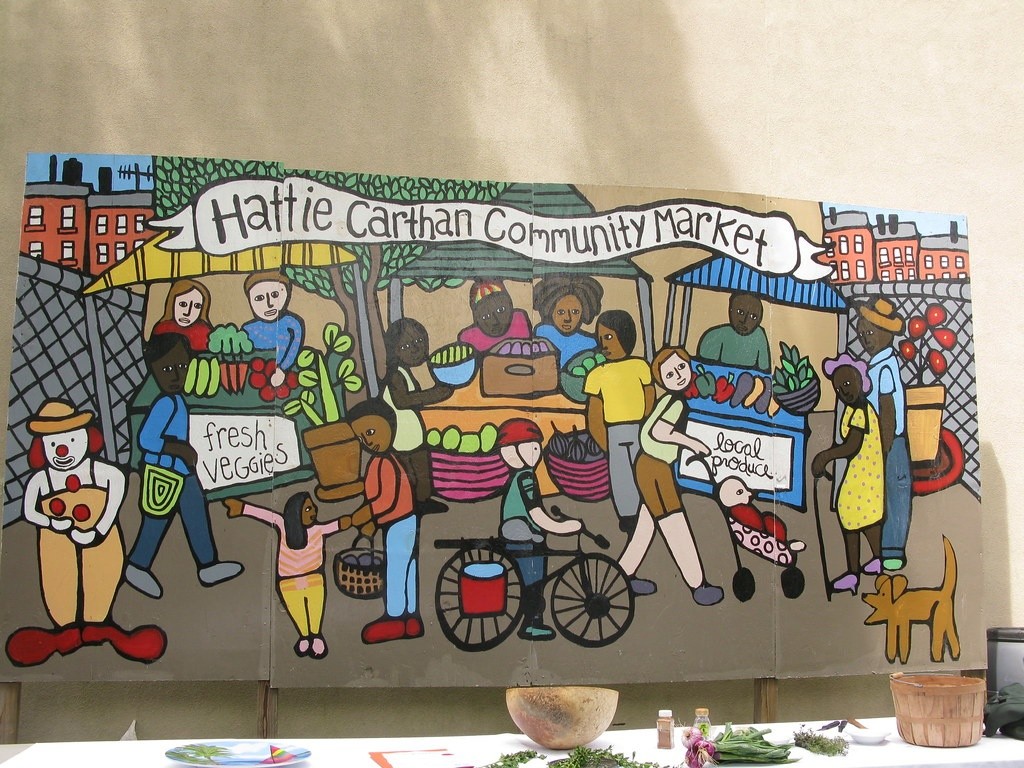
792 724 850 757
471 744 675 768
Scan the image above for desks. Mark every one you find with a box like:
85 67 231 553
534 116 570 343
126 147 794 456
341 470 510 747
1 717 1024 767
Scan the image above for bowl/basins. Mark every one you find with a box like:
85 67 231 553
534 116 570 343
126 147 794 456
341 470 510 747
847 729 890 743
507 686 619 751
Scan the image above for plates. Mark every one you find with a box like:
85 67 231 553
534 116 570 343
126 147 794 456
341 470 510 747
165 741 311 768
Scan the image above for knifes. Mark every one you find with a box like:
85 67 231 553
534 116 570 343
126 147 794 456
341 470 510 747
818 721 840 731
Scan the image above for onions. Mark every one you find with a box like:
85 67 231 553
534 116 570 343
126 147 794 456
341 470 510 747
680 726 715 768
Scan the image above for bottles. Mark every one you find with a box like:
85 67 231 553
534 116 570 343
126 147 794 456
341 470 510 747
694 708 712 740
656 710 675 749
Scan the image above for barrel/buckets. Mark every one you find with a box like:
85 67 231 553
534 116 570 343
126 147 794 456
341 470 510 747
889 672 987 745
